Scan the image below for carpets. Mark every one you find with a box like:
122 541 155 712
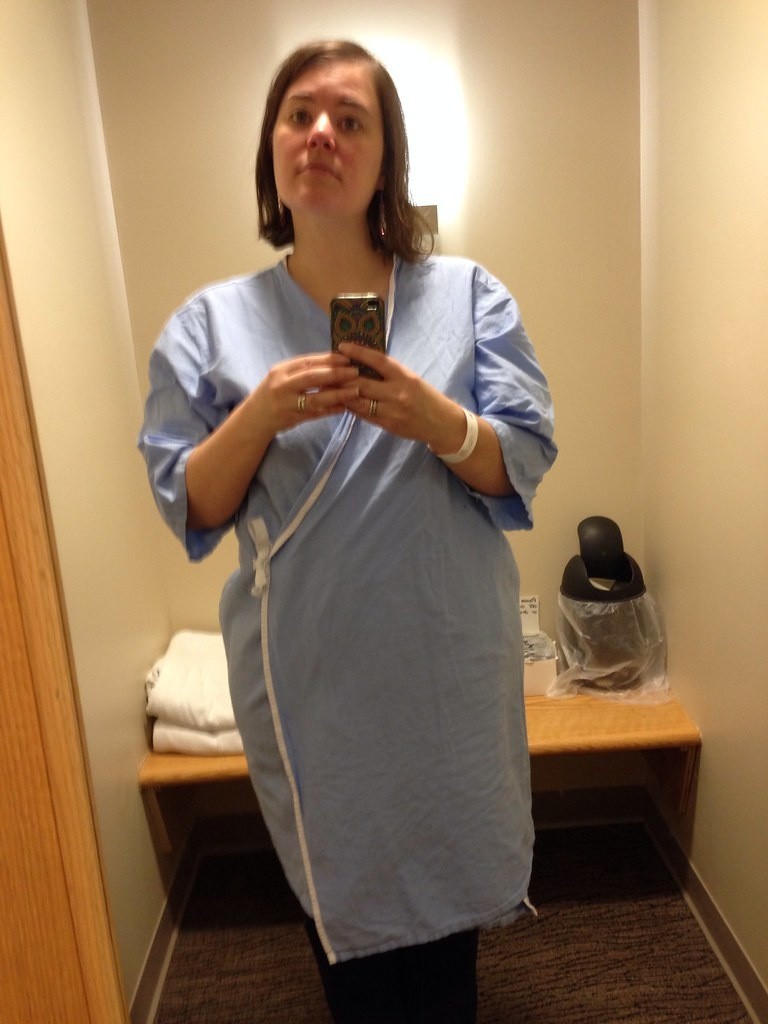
150 820 761 1024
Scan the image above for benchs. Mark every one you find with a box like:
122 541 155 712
129 658 703 855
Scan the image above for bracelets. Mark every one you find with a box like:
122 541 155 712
438 410 479 468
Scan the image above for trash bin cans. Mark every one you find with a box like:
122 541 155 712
557 516 650 692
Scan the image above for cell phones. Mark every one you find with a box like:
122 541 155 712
331 294 386 382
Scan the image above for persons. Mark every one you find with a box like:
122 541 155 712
138 40 559 1024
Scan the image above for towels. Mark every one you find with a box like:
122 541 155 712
144 628 253 756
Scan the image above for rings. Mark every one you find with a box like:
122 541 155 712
297 392 306 413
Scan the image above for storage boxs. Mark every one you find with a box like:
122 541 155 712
520 594 559 694
